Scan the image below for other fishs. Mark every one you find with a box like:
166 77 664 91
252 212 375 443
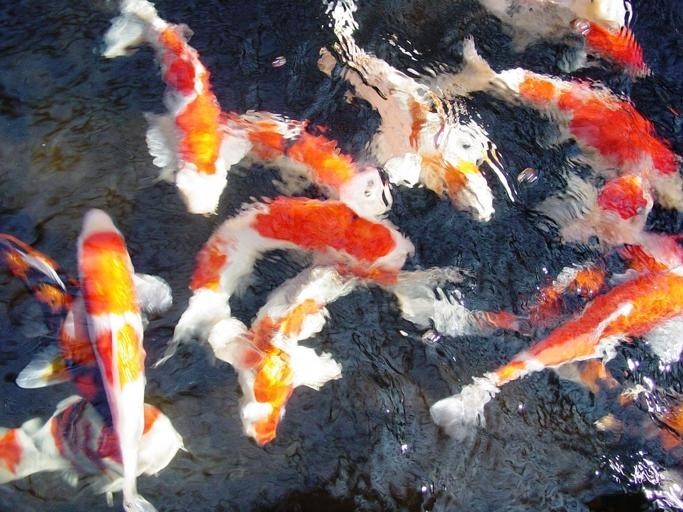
102 0 683 449
